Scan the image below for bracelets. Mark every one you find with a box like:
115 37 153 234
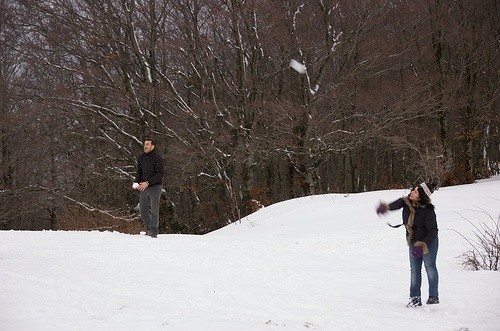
146 181 150 185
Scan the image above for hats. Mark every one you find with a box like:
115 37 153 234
420 182 439 198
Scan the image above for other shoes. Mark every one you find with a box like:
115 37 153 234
145 230 158 238
426 299 440 305
407 298 422 308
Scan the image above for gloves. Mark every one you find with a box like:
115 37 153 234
376 204 388 215
412 246 423 257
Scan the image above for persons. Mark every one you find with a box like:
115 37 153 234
376 181 439 307
132 138 163 239
